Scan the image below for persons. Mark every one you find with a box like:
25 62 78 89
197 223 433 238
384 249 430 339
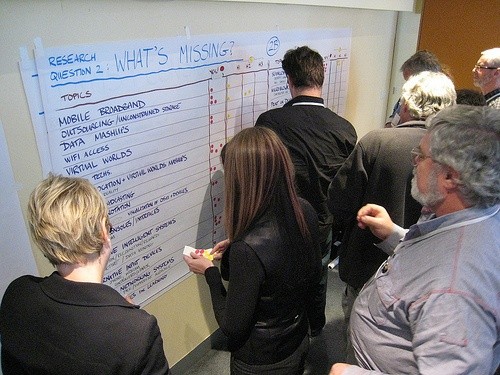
183 45 500 375
0 171 172 375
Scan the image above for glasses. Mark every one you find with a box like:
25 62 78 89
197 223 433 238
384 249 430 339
474 64 498 70
411 146 431 165
